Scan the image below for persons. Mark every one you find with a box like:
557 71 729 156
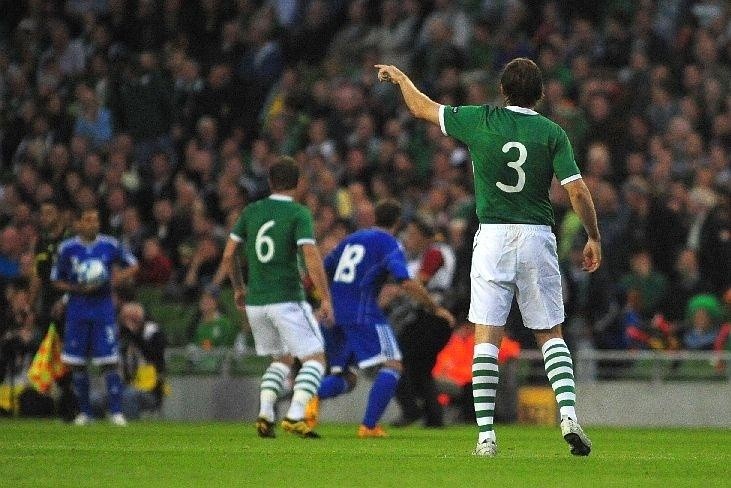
218 155 336 440
388 215 453 429
49 206 141 426
372 58 605 459
278 195 456 439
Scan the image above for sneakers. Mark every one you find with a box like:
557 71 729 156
255 417 276 439
281 416 321 440
389 404 446 429
73 413 127 427
475 437 497 457
305 396 320 429
358 424 389 437
560 413 593 456
